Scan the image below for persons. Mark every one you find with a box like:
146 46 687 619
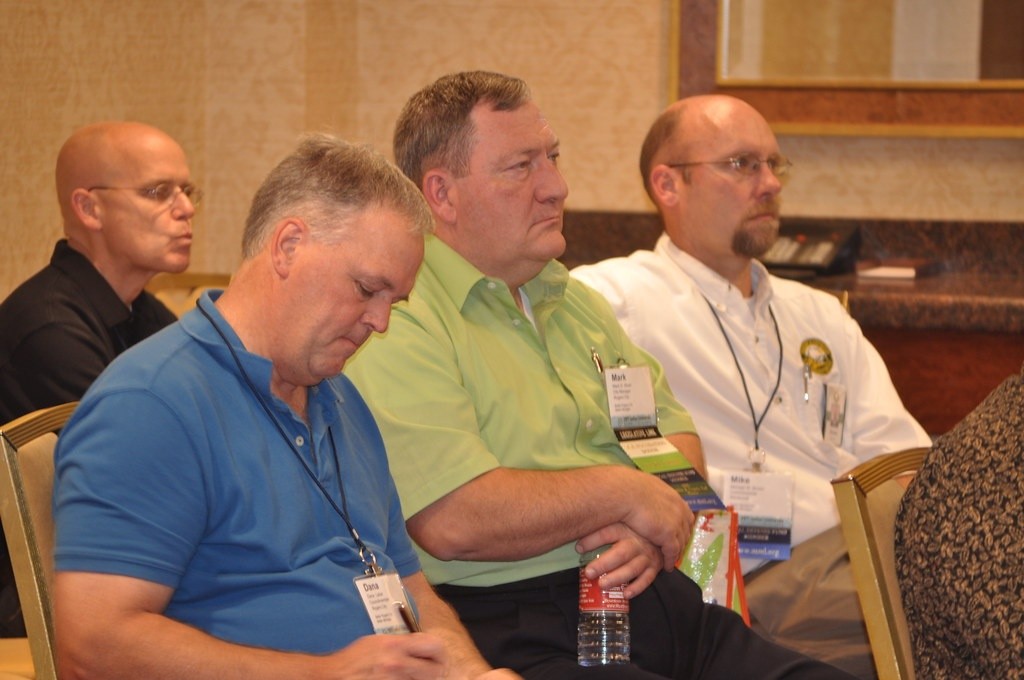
566 96 932 680
826 402 843 422
0 122 205 639
889 371 1024 679
50 135 525 680
337 71 866 680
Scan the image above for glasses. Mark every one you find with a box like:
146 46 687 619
670 152 793 186
88 176 203 214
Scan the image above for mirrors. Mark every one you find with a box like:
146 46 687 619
671 0 1024 139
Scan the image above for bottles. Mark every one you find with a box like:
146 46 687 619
577 552 631 667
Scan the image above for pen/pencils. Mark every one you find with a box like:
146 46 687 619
399 605 418 632
803 364 812 402
591 346 604 372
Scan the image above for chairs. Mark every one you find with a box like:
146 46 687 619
0 401 80 680
830 446 930 680
